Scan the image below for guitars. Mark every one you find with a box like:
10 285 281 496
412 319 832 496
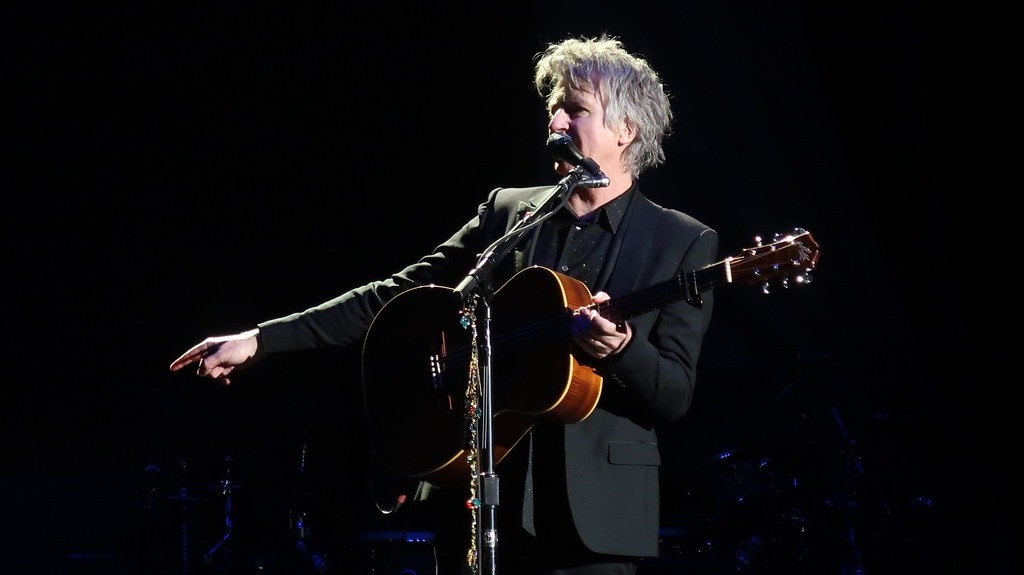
358 216 827 485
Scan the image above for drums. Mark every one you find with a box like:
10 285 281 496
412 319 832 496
695 431 866 553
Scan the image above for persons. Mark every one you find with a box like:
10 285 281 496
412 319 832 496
169 32 722 575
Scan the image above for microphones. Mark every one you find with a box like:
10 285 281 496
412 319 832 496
546 133 610 187
